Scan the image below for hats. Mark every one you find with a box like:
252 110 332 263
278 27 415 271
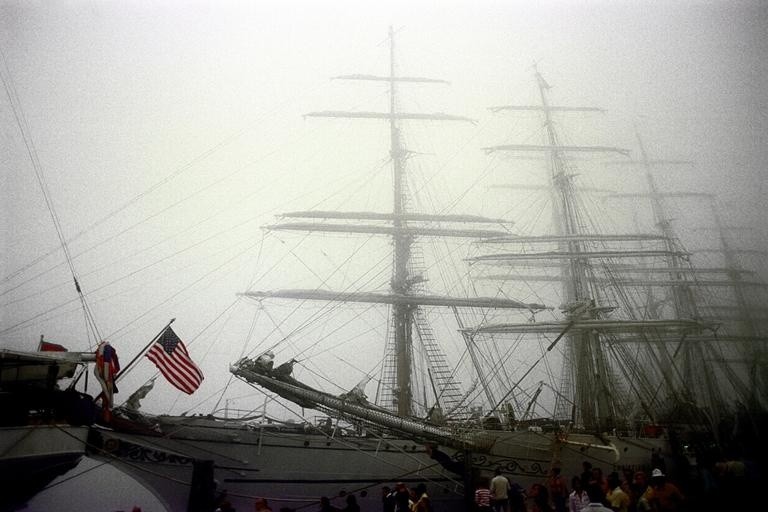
652 468 666 477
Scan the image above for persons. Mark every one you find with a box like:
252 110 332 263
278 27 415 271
462 459 766 510
381 482 434 511
344 493 361 511
254 498 272 511
318 496 341 512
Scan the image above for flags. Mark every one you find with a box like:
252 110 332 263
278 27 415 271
39 341 68 352
146 326 205 395
